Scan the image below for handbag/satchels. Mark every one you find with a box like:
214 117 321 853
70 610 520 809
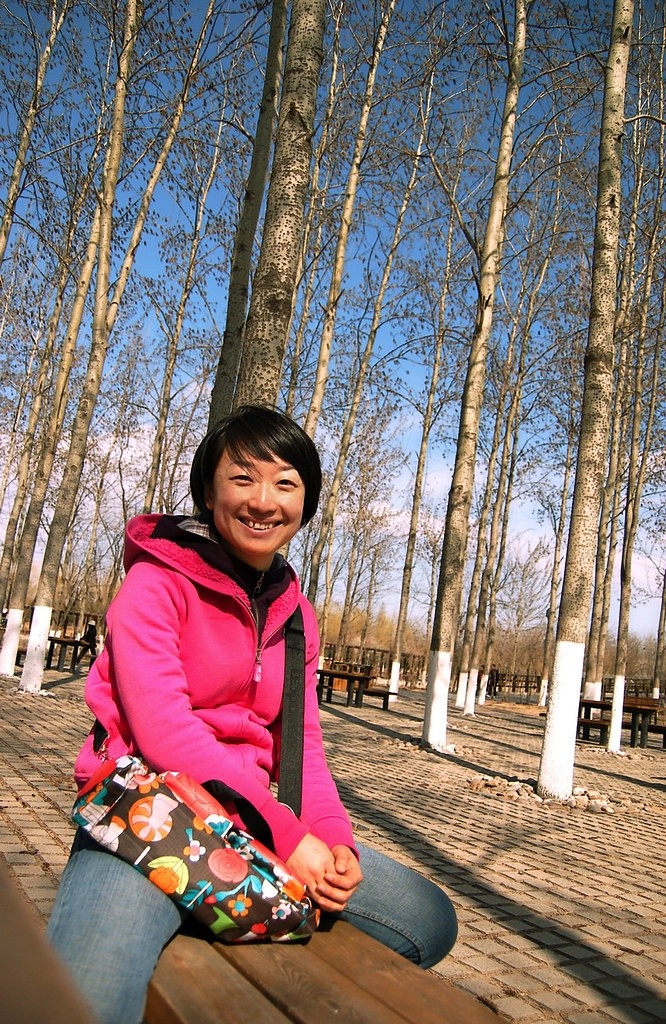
71 754 321 944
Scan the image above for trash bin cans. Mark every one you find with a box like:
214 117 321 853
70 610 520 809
330 662 362 692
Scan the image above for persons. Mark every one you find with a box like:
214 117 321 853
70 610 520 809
475 664 499 698
77 620 97 664
41 403 458 1024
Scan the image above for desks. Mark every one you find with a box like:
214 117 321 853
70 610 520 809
316 669 376 707
45 635 90 673
579 699 658 749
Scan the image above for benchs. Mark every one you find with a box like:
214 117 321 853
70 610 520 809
16 648 27 665
539 712 608 745
144 918 499 1024
355 687 398 710
603 718 666 748
316 684 331 703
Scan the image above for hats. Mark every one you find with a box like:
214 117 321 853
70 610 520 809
88 620 95 626
